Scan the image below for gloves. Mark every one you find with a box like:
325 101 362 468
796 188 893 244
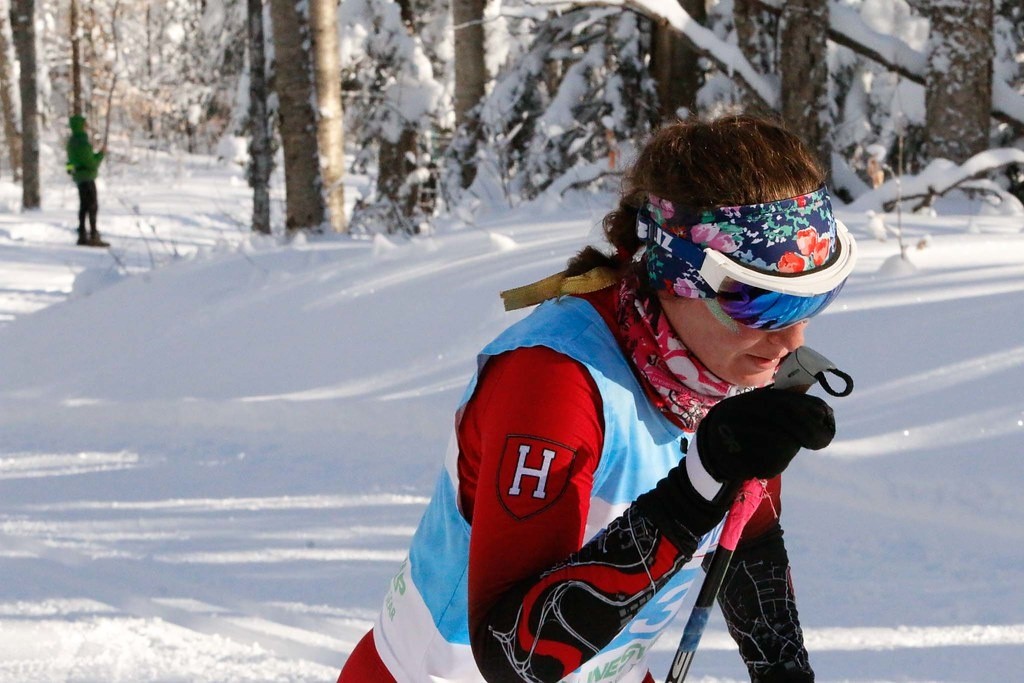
649 388 837 537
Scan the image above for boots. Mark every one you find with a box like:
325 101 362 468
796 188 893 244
79 230 111 246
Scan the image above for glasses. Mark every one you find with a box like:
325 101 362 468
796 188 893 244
696 220 856 333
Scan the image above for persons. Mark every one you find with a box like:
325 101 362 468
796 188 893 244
66 115 111 247
335 114 857 682
